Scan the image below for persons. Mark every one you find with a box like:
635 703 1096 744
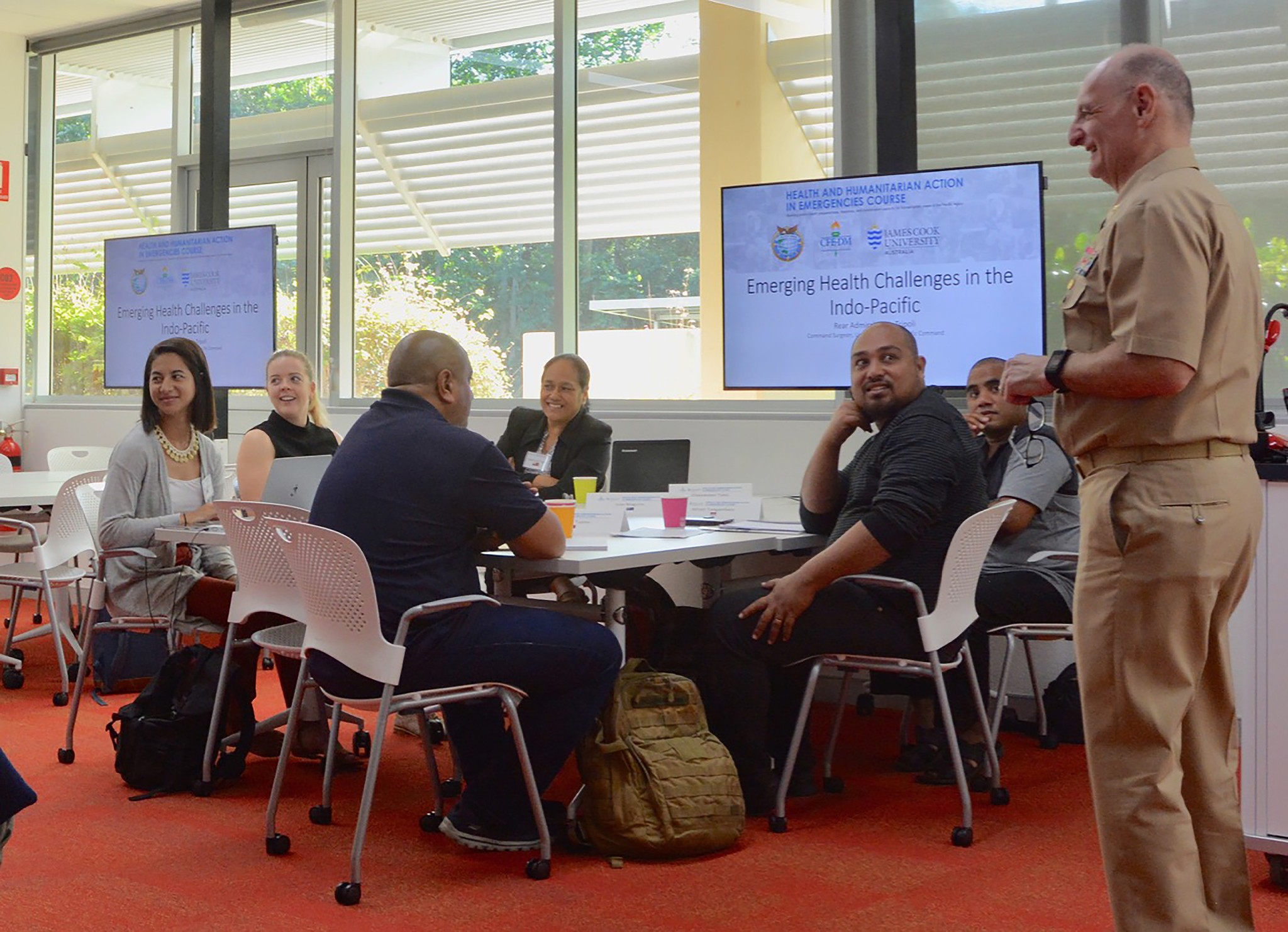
898 357 1080 785
997 44 1266 932
237 349 450 743
308 329 622 849
484 354 612 604
701 324 990 817
98 339 364 769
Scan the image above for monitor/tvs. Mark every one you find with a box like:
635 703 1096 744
721 161 1046 391
104 224 277 388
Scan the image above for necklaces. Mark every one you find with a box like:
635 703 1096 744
153 421 202 461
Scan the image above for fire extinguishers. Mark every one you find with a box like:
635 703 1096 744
0 419 29 472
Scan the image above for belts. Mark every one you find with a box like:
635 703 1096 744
1078 438 1242 478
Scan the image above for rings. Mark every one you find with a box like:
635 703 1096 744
775 618 780 621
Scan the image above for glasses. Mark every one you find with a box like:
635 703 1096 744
1008 396 1046 467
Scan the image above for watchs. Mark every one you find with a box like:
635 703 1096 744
1045 349 1074 394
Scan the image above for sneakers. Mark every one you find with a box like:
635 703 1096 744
393 712 448 741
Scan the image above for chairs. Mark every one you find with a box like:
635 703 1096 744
768 499 1079 846
0 439 552 907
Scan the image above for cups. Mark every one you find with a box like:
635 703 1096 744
573 477 598 508
661 495 688 528
544 499 575 539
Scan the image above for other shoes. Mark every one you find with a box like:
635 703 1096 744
439 795 566 851
916 739 1004 785
745 765 816 815
896 742 944 772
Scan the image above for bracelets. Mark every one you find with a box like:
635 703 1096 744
183 511 187 527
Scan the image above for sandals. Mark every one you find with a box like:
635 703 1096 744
291 727 363 772
222 720 288 757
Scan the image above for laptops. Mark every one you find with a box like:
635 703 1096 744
610 439 692 493
260 455 334 512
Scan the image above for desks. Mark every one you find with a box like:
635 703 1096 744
0 471 105 663
156 516 776 847
776 523 829 552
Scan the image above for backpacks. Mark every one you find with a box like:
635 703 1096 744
105 643 255 801
1042 662 1085 743
567 659 746 868
